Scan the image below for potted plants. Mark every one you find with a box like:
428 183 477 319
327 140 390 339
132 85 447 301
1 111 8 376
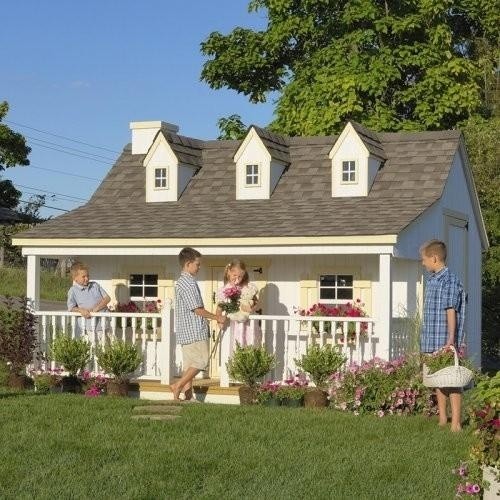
0 294 142 398
223 340 348 409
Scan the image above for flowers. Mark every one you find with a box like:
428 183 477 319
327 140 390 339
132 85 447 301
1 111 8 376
210 283 242 361
298 300 369 337
110 298 159 327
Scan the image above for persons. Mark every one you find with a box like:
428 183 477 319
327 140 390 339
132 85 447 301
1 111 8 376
420 237 469 431
216 259 262 368
66 262 120 371
168 247 227 403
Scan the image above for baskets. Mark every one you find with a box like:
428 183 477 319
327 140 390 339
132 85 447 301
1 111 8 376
422 345 473 389
